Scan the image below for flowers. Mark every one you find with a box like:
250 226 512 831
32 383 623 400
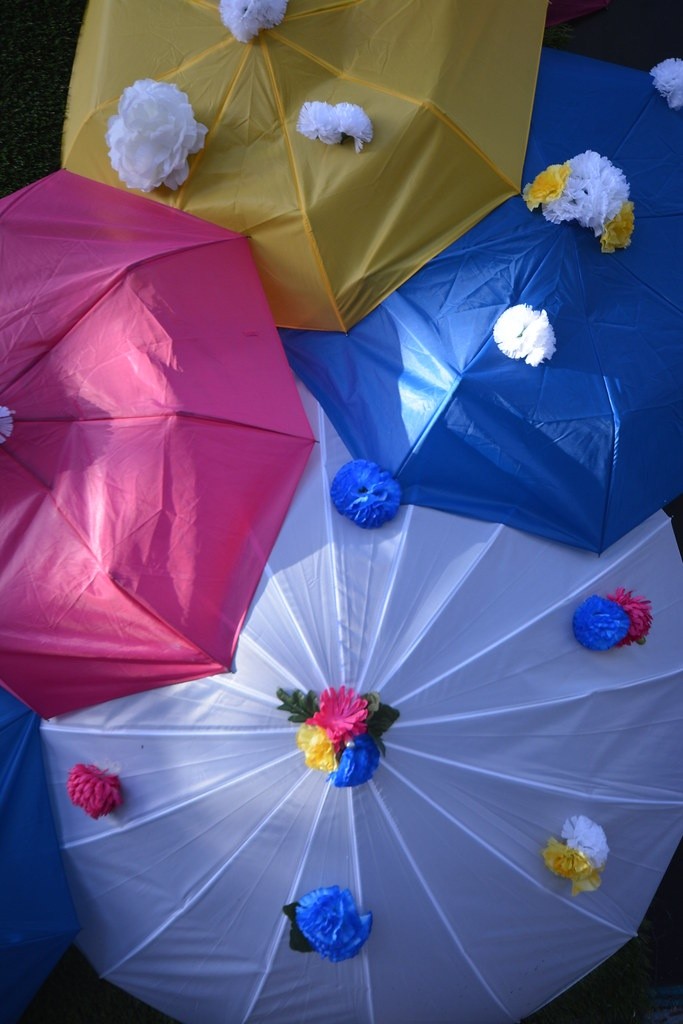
542 837 606 896
606 587 653 648
493 304 557 368
523 163 573 213
325 735 379 789
600 201 635 253
104 78 209 193
649 58 683 111
542 150 630 237
296 100 342 145
295 722 338 773
219 0 289 44
304 685 368 752
330 102 373 153
65 763 125 821
561 815 610 868
572 595 630 652
330 460 401 530
296 885 372 964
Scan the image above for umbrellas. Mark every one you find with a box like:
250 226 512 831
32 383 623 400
2 3 683 1017
